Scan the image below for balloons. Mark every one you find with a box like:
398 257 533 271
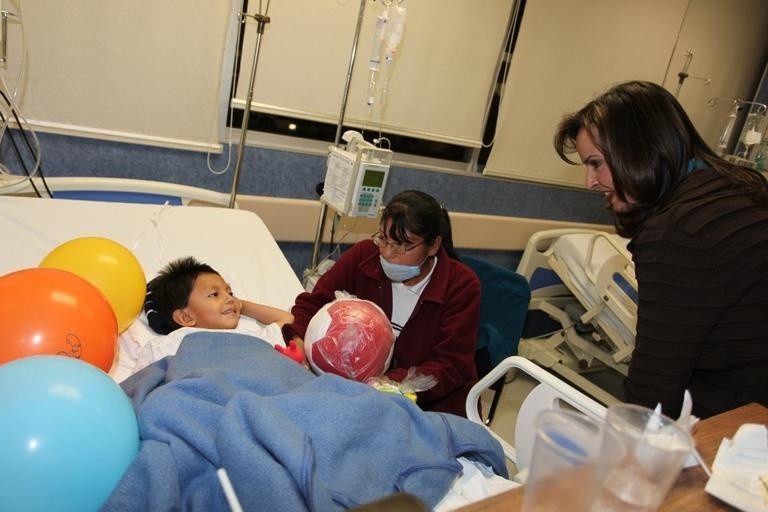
40 236 150 336
0 353 140 511
0 267 119 373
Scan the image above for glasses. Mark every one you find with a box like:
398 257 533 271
371 231 425 256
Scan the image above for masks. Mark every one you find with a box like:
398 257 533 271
380 256 428 282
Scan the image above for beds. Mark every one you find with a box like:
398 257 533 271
0 177 644 512
516 226 638 407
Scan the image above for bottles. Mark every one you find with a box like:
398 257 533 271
384 8 406 62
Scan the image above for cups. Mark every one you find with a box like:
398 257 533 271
523 403 696 510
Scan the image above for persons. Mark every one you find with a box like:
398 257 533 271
136 256 295 350
551 80 767 427
280 190 485 423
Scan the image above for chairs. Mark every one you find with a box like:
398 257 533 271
459 253 531 425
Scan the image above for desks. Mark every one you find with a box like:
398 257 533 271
455 401 768 512
